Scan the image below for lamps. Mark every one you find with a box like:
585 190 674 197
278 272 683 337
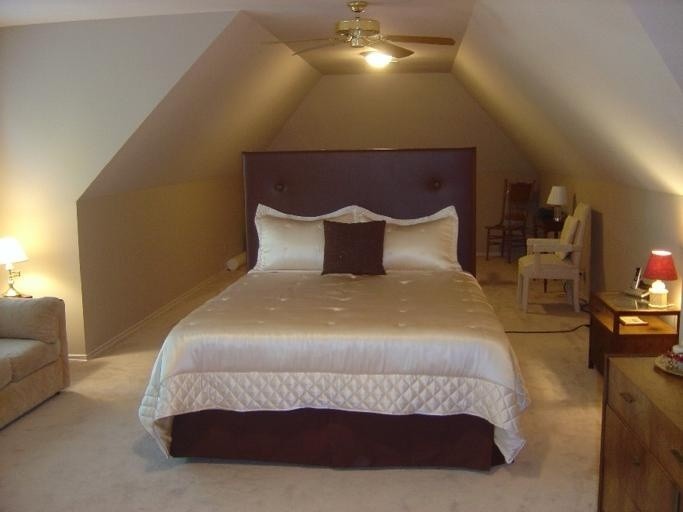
642 248 681 309
359 51 401 70
0 234 30 298
546 184 567 225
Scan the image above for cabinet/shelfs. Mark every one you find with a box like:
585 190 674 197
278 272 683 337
585 287 682 377
594 352 682 511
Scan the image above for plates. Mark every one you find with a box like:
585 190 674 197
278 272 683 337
655 355 683 378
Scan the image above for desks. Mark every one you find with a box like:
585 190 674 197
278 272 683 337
531 221 566 296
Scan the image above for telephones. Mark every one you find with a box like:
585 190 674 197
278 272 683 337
623 266 652 299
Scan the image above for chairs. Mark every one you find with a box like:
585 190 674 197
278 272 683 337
0 291 73 435
483 176 536 265
515 200 591 317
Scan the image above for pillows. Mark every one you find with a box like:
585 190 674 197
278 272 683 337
243 200 355 274
317 217 388 275
349 204 465 273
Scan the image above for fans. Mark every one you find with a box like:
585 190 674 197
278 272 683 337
251 0 456 61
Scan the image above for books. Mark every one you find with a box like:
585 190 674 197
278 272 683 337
619 315 649 326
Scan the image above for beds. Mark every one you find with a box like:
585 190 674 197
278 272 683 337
131 145 530 476
